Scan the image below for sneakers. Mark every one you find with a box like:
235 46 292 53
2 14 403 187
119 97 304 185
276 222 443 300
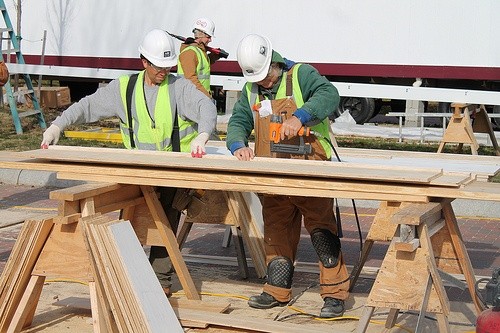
248 291 290 309
320 297 346 318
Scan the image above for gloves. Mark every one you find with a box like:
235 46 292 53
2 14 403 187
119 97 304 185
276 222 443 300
41 124 60 148
212 47 229 59
190 131 210 157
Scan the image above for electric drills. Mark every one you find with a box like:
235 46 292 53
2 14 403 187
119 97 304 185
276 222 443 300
269 114 313 158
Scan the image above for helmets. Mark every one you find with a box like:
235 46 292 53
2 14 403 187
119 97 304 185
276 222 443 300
236 33 273 83
192 17 215 37
139 29 179 68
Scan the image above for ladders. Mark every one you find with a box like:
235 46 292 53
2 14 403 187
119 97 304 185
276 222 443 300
385 112 500 146
0 0 47 135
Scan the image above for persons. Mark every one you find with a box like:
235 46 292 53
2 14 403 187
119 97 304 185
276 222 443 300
227 33 351 318
41 29 217 296
176 18 223 141
